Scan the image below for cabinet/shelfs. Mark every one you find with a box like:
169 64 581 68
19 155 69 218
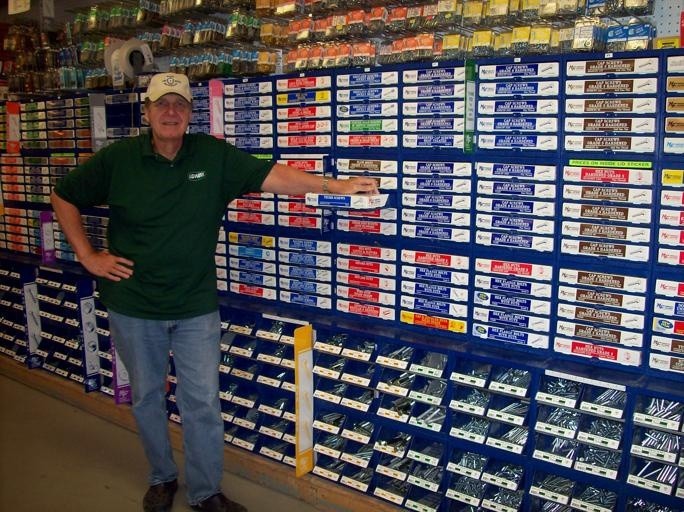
1 48 684 512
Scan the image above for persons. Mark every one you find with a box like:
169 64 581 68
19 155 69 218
52 71 378 510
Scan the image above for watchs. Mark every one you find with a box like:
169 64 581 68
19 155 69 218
321 175 332 195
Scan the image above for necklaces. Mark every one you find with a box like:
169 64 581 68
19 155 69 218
153 134 180 161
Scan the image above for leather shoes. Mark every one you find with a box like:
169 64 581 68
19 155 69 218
190 493 248 511
142 478 177 512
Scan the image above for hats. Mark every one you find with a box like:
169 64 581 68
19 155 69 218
143 73 192 103
